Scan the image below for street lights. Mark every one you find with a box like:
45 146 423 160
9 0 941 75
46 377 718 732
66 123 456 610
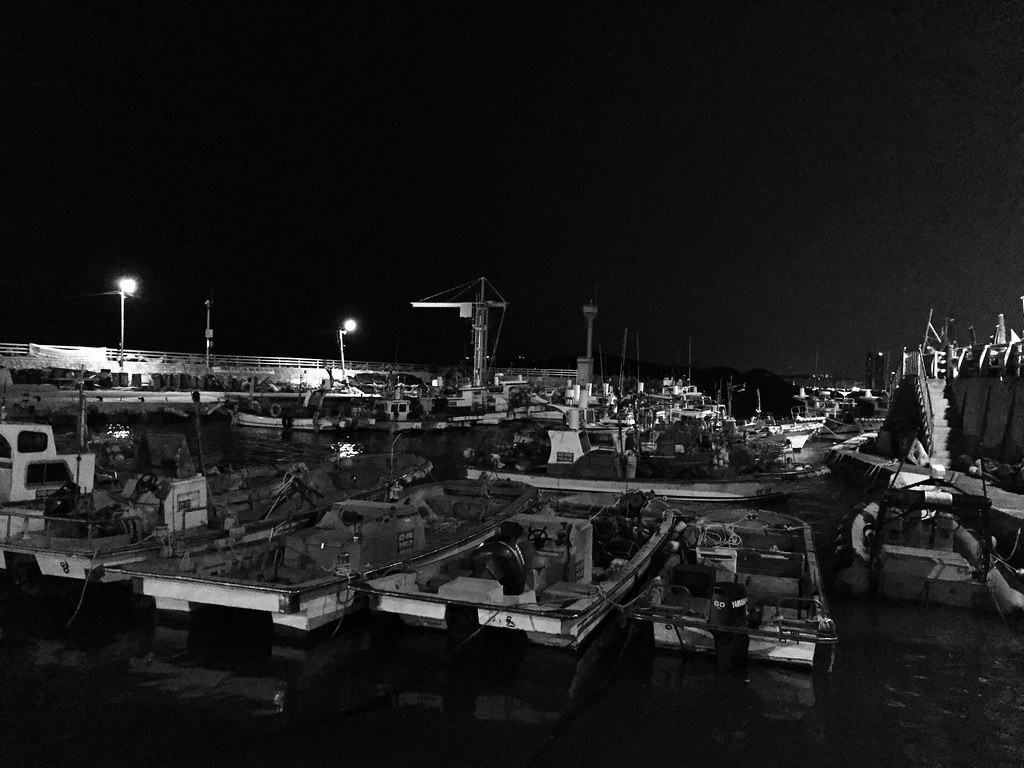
118 277 137 368
339 320 357 374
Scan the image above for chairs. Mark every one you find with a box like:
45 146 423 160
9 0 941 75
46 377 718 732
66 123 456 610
662 566 720 618
481 535 526 597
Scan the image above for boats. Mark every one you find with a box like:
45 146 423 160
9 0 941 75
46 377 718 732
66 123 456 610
500 379 576 421
0 424 310 541
852 477 1024 622
0 452 434 583
346 492 679 653
102 473 541 650
229 377 885 501
635 508 839 673
750 422 858 464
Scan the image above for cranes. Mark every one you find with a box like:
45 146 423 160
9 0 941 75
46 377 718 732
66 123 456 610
410 276 507 385
